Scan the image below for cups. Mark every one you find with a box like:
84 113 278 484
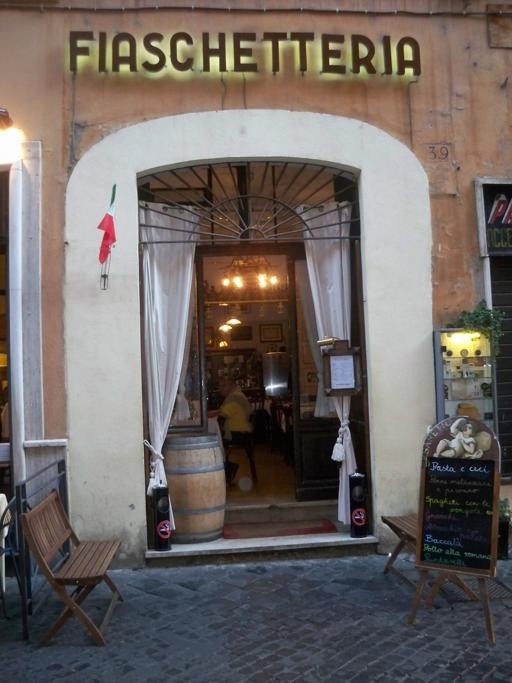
484 412 493 418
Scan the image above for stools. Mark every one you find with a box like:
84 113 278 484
226 439 258 489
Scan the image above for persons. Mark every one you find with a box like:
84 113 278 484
432 416 476 458
207 373 255 484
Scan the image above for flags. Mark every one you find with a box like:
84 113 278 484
97 182 116 263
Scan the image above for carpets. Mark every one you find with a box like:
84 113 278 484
223 517 337 538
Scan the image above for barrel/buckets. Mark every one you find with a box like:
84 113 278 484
162 435 226 543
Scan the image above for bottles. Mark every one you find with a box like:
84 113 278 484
456 403 475 417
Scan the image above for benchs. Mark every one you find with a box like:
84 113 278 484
22 486 124 647
380 505 480 607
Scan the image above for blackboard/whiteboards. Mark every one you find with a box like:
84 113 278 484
414 456 500 578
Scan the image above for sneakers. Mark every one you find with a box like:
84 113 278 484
226 463 239 482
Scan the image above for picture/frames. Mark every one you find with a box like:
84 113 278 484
259 322 284 343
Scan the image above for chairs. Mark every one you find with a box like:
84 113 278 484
1 494 24 620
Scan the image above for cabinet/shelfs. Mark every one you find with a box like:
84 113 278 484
432 326 501 439
